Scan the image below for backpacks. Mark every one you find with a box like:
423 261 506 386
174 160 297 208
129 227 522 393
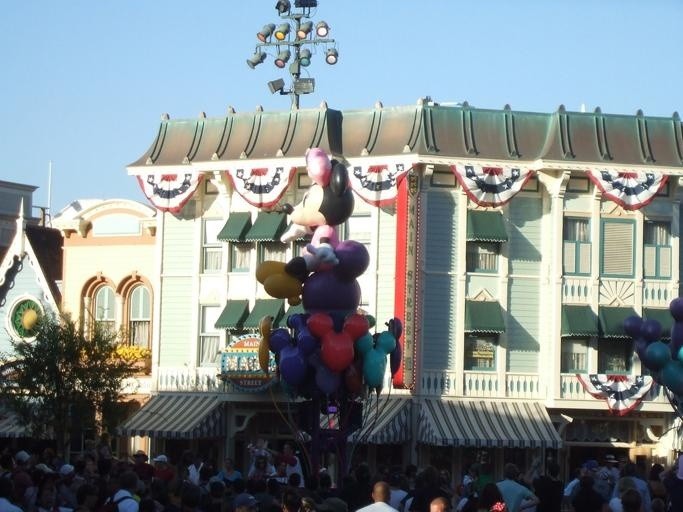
98 489 134 512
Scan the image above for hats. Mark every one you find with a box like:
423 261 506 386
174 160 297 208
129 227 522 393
60 464 75 475
604 454 619 463
133 450 149 462
16 450 30 462
232 494 261 512
152 455 168 463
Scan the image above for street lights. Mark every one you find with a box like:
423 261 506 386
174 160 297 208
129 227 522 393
245 1 339 110
